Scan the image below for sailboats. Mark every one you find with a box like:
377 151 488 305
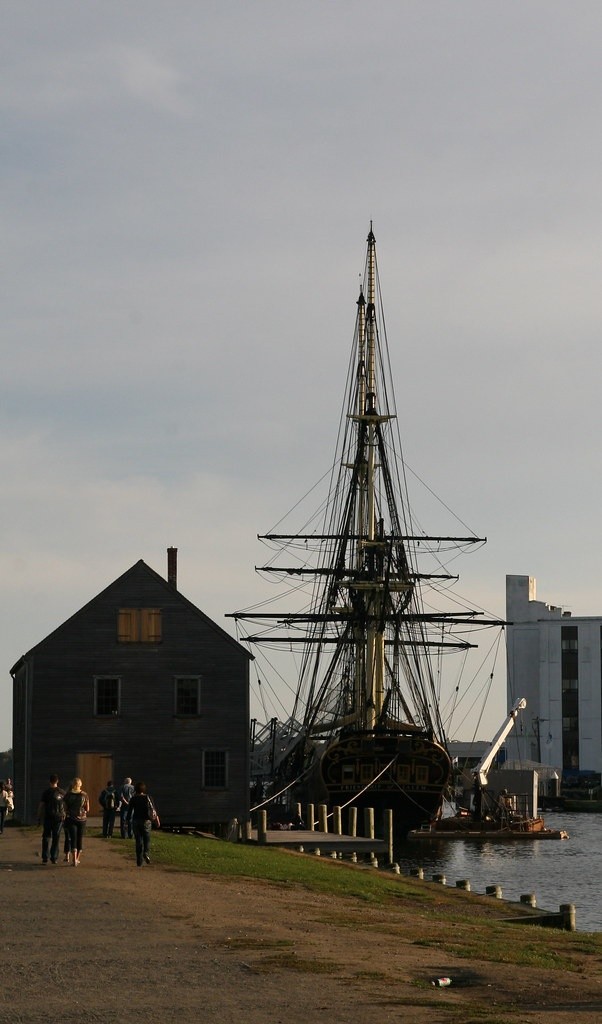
225 219 516 841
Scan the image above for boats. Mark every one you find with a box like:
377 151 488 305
409 698 568 842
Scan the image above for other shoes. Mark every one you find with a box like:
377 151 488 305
52 861 58 864
40 862 47 866
63 859 69 863
144 853 151 864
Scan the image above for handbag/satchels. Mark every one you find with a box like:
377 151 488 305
147 798 157 820
4 791 15 812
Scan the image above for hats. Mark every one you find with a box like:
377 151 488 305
51 774 59 780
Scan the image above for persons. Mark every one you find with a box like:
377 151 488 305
37 774 89 868
98 778 137 840
0 778 15 834
126 782 161 867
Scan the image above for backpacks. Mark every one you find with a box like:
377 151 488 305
48 788 65 822
104 789 117 810
65 792 87 822
